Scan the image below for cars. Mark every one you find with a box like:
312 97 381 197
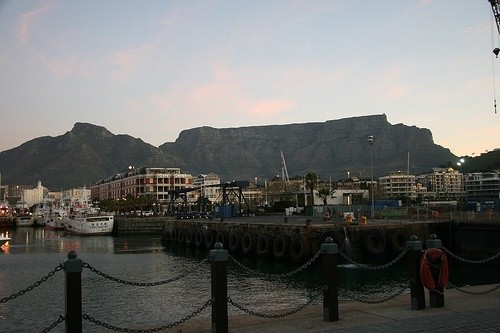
137 211 153 217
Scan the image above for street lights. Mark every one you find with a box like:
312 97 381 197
457 158 465 211
367 134 375 222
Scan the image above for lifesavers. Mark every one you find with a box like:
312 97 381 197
388 227 410 254
160 232 167 241
272 235 290 260
215 230 228 248
186 230 193 246
195 230 203 247
205 230 213 247
362 229 386 256
241 233 253 254
291 234 310 261
170 228 176 242
229 233 239 253
420 248 448 290
178 229 185 242
256 232 272 258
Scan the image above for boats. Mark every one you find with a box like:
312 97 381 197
0 234 12 248
62 203 114 236
0 197 68 231
